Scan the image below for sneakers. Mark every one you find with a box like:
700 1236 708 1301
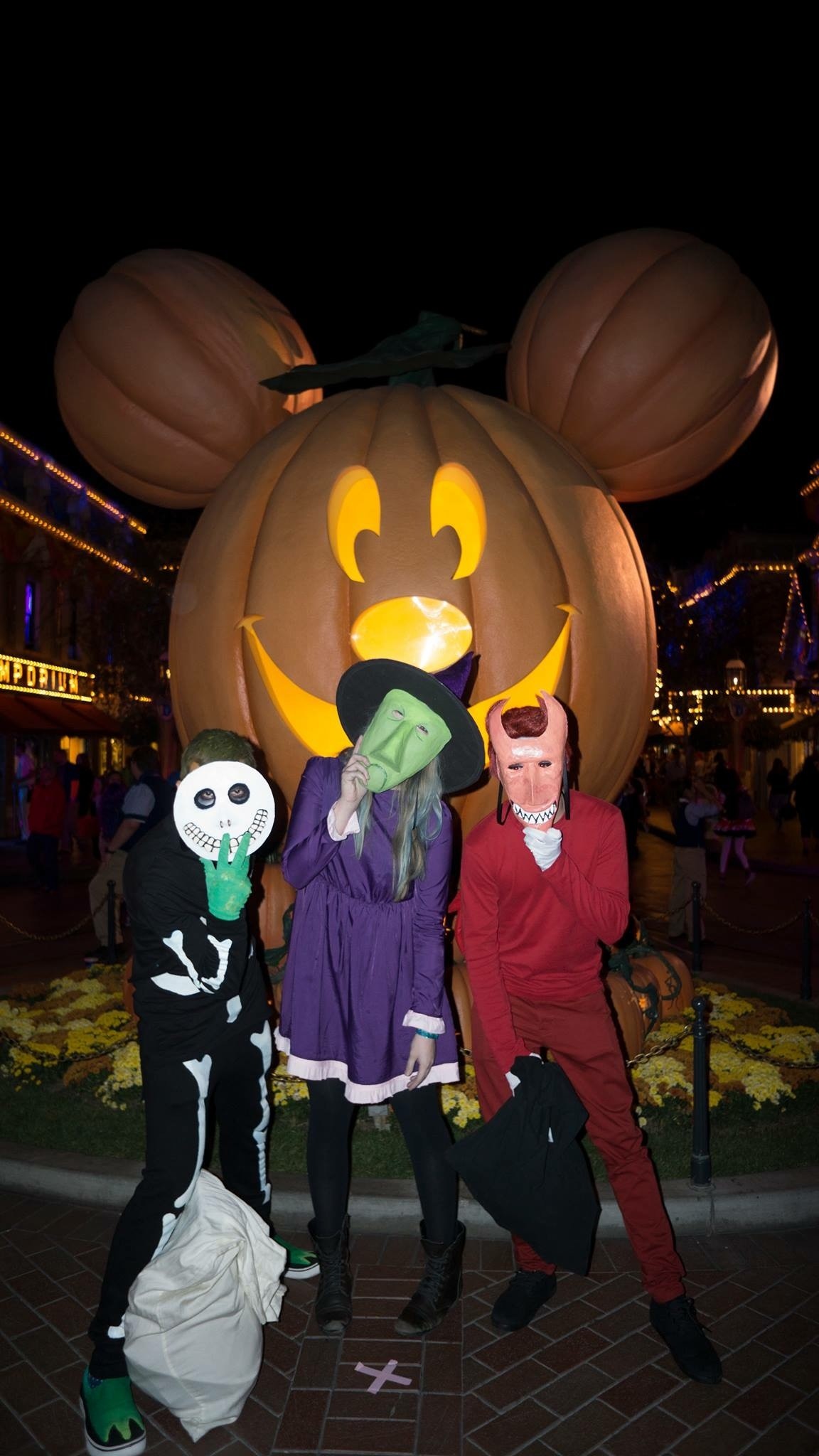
78 1365 146 1455
265 1232 320 1279
490 1266 557 1333
647 1296 723 1384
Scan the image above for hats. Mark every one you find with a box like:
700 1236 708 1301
335 650 484 794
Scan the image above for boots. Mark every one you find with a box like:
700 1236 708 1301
391 1217 466 1338
307 1213 353 1335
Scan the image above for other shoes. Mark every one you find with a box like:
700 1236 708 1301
801 847 809 856
668 932 689 943
110 944 129 962
82 945 112 966
744 873 757 889
718 879 728 889
688 939 719 951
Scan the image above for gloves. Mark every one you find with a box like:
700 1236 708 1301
522 827 562 870
199 831 253 920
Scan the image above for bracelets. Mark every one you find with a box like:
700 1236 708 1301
415 1027 439 1041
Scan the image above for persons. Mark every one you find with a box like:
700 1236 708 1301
457 704 728 1388
71 725 323 1456
280 665 466 1338
626 729 819 954
1 723 163 967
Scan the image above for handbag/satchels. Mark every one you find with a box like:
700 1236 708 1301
124 1168 285 1435
443 1054 600 1278
